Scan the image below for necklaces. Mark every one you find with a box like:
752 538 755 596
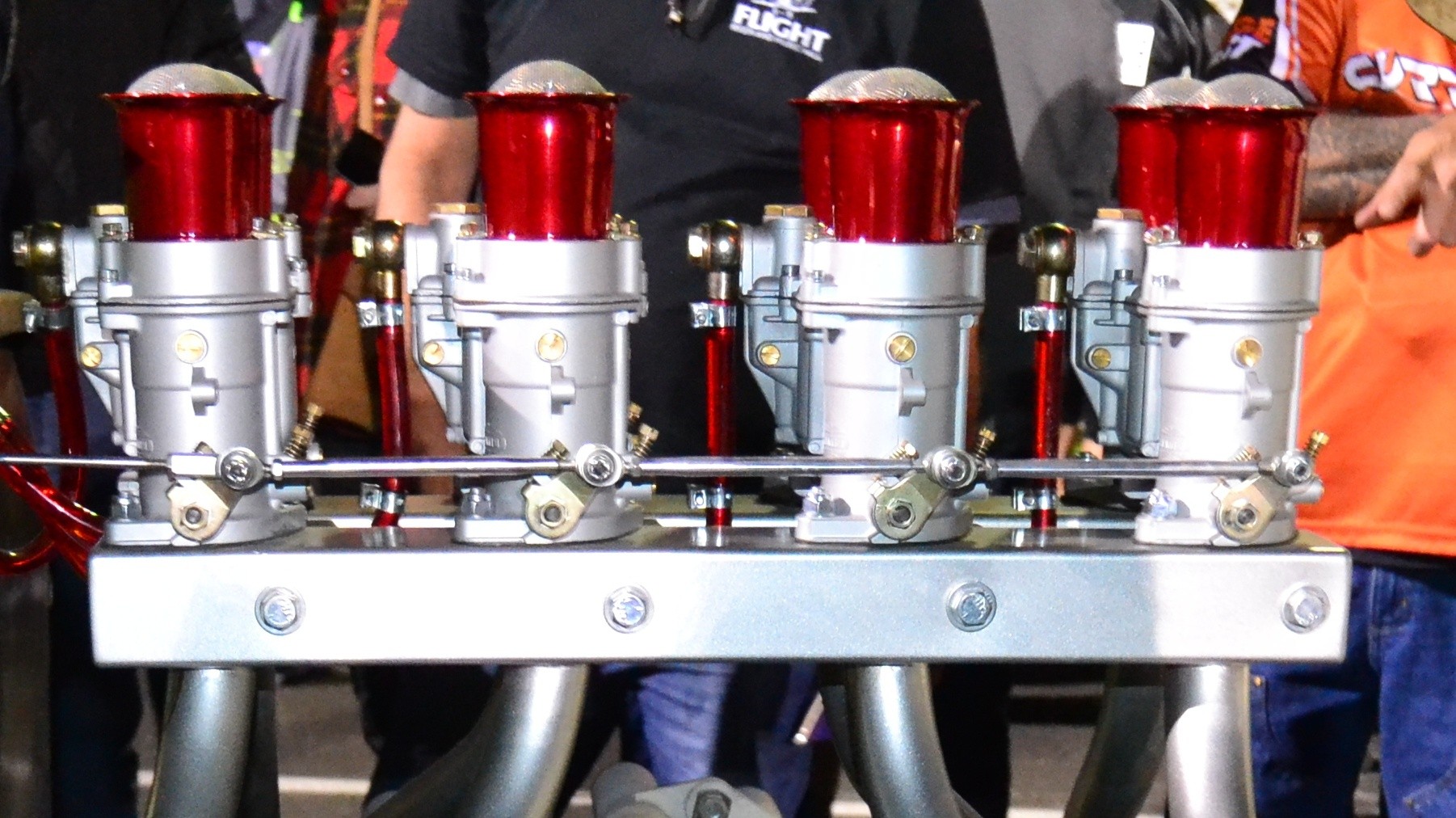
664 1 687 23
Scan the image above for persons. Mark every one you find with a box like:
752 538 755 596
0 1 1454 818
